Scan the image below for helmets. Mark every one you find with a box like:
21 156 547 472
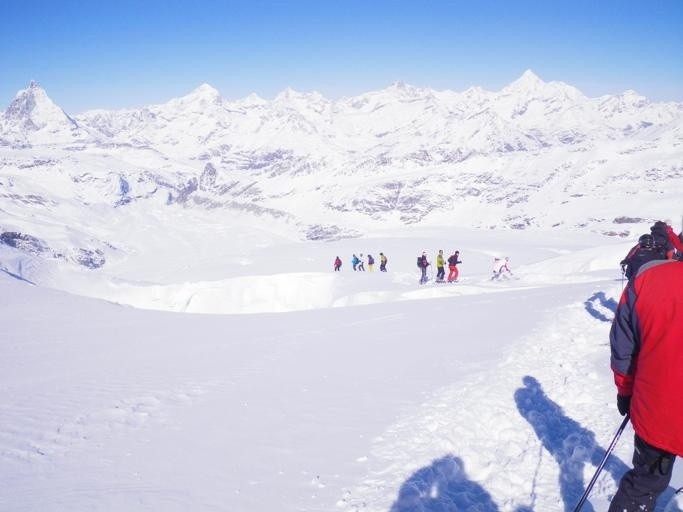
640 235 653 248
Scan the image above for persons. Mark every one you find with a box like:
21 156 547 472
488 256 515 281
367 255 375 272
606 220 683 512
447 250 462 284
435 249 447 283
379 252 388 272
334 256 342 271
359 253 365 271
351 254 359 272
417 251 431 285
